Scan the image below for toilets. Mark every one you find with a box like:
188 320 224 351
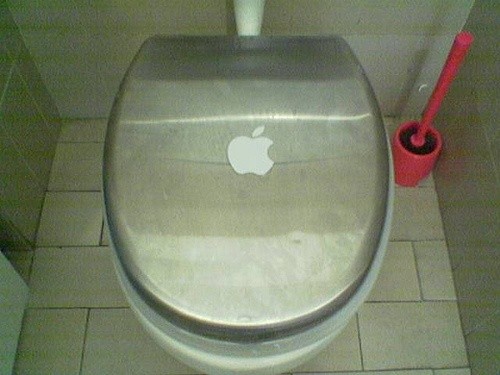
103 34 394 372
